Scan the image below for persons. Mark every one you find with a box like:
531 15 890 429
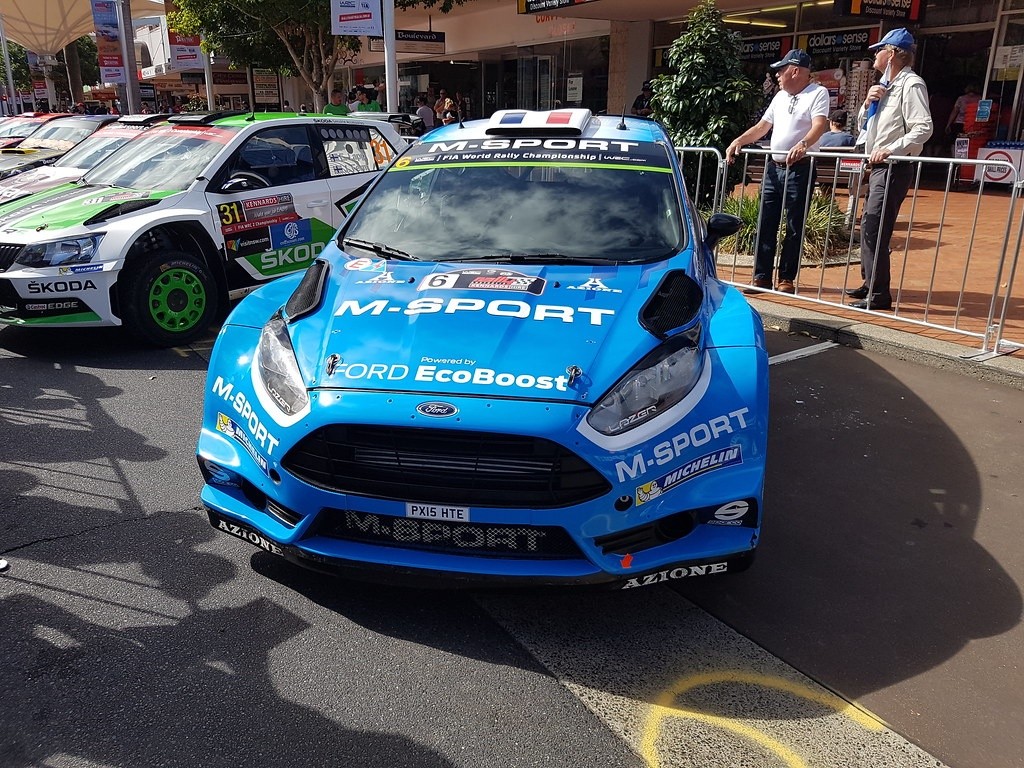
416 97 434 135
284 101 293 112
944 81 982 187
816 110 856 201
726 49 829 293
95 102 120 114
140 95 187 113
323 81 385 115
631 80 656 116
241 101 249 110
300 104 314 112
36 98 92 114
844 27 933 309
434 89 467 127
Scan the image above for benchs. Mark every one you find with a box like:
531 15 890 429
746 145 869 197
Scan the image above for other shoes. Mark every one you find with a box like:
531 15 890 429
777 277 794 294
845 285 869 299
848 299 892 309
742 280 773 295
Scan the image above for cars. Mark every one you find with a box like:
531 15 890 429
0 101 425 347
194 104 772 594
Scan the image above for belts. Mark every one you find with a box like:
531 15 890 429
771 157 811 168
869 159 911 169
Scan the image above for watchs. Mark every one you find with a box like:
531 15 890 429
800 139 808 148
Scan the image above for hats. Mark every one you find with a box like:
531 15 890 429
770 48 812 69
828 110 848 123
867 27 917 54
642 82 654 92
